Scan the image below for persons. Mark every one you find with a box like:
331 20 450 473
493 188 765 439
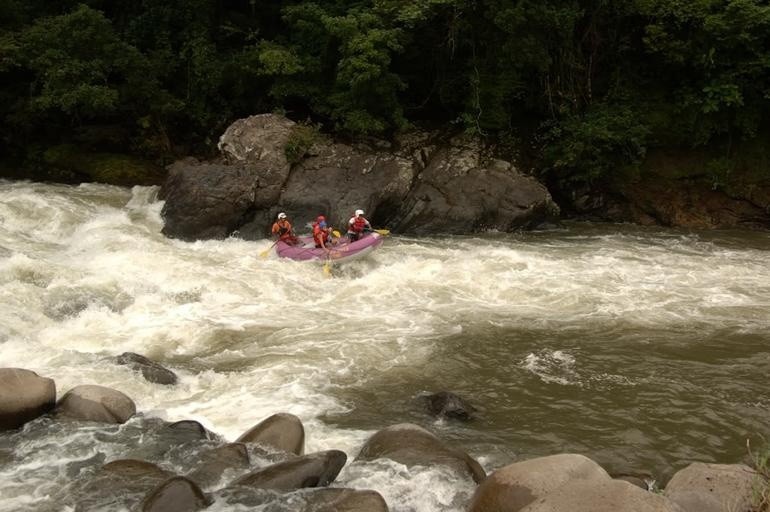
316 222 336 249
347 209 372 242
272 212 299 248
312 216 326 248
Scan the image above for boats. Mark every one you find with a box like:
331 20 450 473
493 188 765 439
276 230 386 265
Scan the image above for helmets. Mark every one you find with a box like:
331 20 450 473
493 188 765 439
355 209 364 218
317 216 327 228
277 213 287 219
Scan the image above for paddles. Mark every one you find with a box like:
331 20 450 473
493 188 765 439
260 240 278 258
364 228 390 235
333 230 341 238
324 255 330 273
